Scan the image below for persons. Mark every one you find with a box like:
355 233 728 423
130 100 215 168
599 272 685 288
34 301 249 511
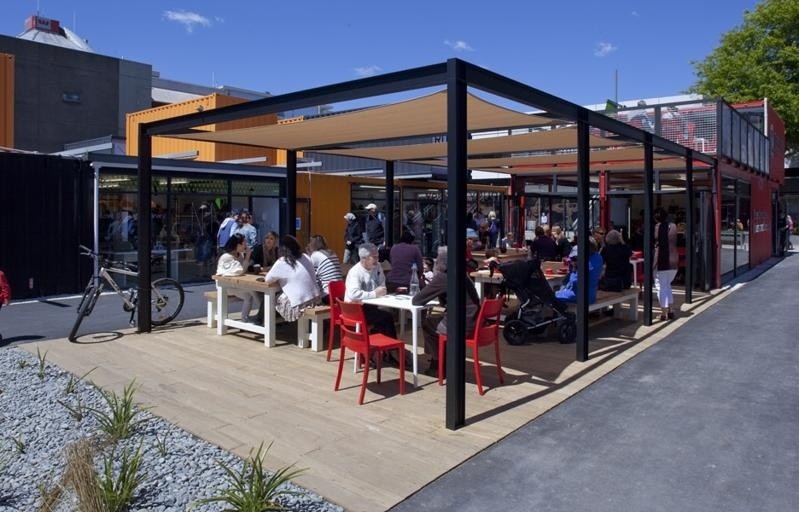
551 236 602 305
345 242 399 367
364 204 387 249
343 212 363 264
385 230 433 293
530 223 573 262
785 213 794 251
590 224 634 291
412 245 481 378
736 217 744 246
661 102 682 136
652 207 680 320
216 211 344 328
101 195 210 272
626 101 655 134
0 271 11 341
466 210 522 279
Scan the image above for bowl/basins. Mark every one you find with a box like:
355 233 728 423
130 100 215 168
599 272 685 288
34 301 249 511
560 268 567 273
396 286 408 294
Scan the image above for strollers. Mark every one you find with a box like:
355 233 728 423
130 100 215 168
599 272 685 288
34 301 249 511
484 256 577 346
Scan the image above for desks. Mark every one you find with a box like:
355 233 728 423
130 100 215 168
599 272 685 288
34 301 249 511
471 248 537 269
354 291 440 387
630 256 646 287
211 269 283 348
469 270 568 322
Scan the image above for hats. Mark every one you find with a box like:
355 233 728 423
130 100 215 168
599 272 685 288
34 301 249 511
365 204 377 209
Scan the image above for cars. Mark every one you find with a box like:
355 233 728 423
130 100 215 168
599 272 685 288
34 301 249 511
103 210 209 250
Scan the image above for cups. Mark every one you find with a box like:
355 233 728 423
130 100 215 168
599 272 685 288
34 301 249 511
253 264 262 275
546 269 553 275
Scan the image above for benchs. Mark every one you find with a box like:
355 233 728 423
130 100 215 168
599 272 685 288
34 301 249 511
202 290 244 330
565 287 641 326
297 306 331 353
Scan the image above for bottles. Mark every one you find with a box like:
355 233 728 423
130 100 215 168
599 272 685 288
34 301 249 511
409 262 420 296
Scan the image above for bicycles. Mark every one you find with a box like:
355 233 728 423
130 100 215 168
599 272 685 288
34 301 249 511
65 240 187 342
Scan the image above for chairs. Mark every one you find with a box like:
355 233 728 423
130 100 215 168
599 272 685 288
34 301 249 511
438 295 506 395
327 279 345 362
333 297 406 405
